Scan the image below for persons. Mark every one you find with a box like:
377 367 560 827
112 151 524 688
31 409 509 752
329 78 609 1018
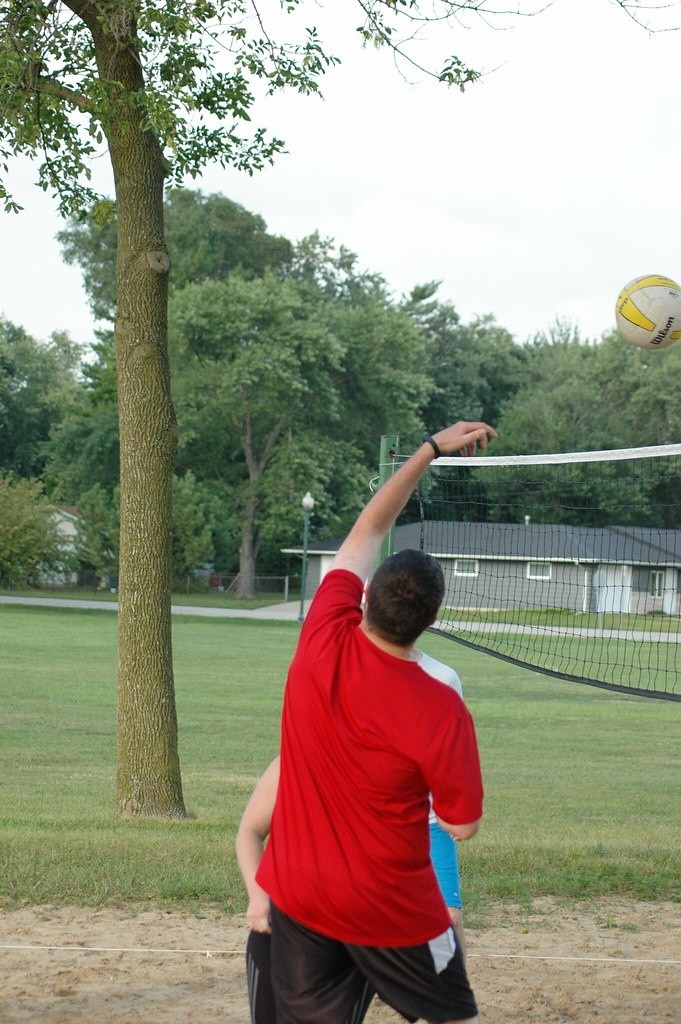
234 421 498 1024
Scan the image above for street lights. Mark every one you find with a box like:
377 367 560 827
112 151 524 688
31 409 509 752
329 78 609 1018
297 490 316 622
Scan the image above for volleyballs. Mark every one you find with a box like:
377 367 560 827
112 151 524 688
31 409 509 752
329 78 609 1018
615 274 681 349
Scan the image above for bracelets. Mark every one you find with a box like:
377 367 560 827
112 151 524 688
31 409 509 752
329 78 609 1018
422 435 441 459
